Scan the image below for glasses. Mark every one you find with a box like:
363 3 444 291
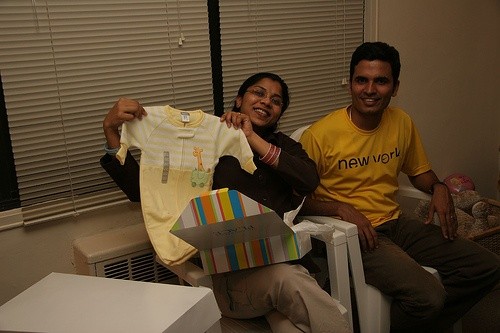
241 90 284 108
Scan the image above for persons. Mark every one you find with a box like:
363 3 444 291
101 72 353 333
293 42 500 333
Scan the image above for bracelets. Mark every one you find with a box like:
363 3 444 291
430 182 448 193
259 143 281 165
104 142 121 155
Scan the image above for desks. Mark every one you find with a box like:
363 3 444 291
0 271 222 333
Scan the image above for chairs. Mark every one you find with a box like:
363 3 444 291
154 220 354 333
290 124 462 332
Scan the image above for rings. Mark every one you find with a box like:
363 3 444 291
451 216 453 218
237 112 241 115
